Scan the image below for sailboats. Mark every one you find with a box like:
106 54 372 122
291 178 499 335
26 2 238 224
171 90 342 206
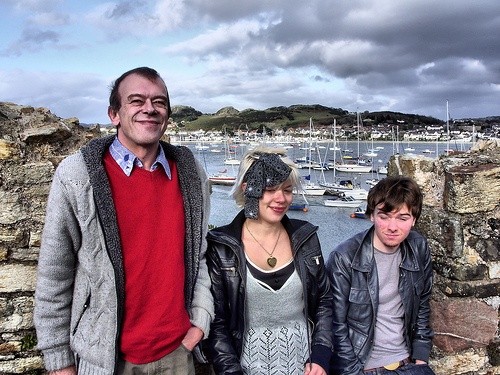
170 99 455 208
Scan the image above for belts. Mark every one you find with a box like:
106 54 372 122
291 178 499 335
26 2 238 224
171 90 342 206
363 355 411 371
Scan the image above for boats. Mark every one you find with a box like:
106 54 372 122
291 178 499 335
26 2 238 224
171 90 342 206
290 202 309 212
210 149 221 153
349 201 370 220
423 149 435 155
209 176 237 186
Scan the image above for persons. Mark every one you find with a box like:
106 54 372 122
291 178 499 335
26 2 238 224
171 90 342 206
206 144 339 375
326 175 434 375
33 66 216 375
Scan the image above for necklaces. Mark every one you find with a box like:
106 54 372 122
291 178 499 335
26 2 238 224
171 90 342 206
243 219 285 268
